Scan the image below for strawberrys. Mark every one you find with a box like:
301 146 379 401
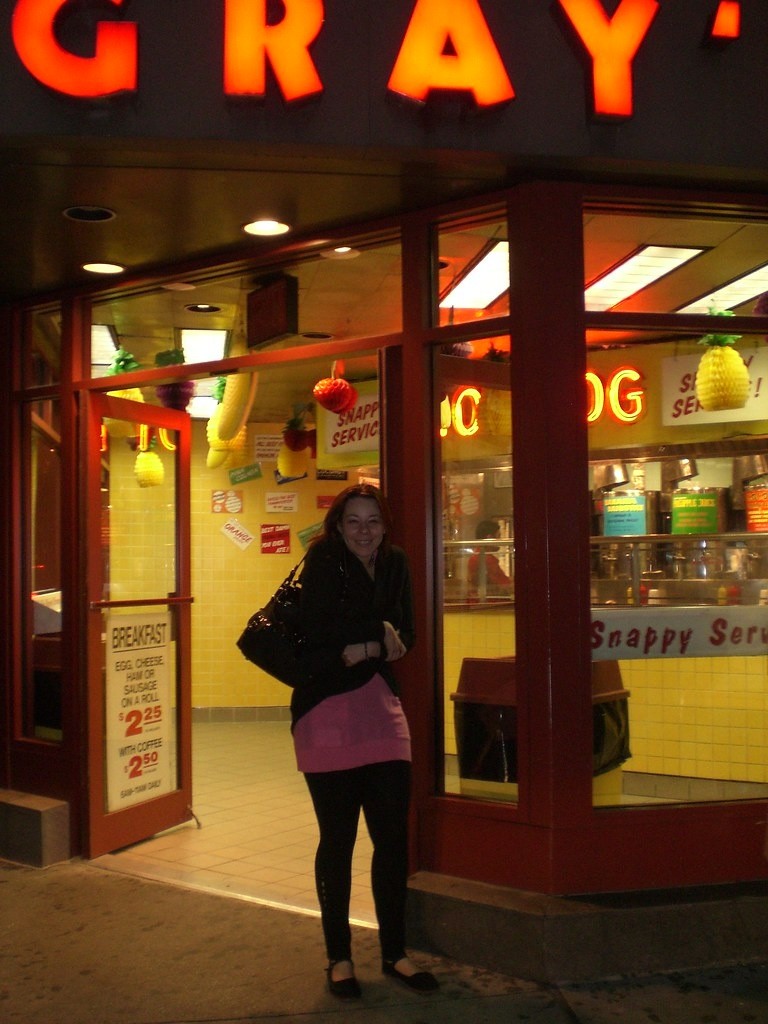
312 377 358 416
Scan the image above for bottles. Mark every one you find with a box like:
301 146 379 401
718 585 726 606
627 587 633 605
640 584 646 604
729 584 736 604
760 587 768 605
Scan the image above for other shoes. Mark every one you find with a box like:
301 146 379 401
380 957 439 996
325 958 361 1004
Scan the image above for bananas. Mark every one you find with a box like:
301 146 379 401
217 334 258 441
205 447 228 469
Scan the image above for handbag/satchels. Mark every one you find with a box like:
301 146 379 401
237 538 348 688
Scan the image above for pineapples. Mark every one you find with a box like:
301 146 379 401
206 376 249 451
277 445 310 478
104 349 145 440
134 434 164 488
696 310 751 410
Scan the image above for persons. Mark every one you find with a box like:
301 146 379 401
468 521 514 604
290 483 440 1001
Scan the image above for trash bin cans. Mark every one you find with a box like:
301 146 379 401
448 656 636 806
31 628 106 743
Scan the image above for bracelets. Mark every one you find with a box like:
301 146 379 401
364 642 369 661
